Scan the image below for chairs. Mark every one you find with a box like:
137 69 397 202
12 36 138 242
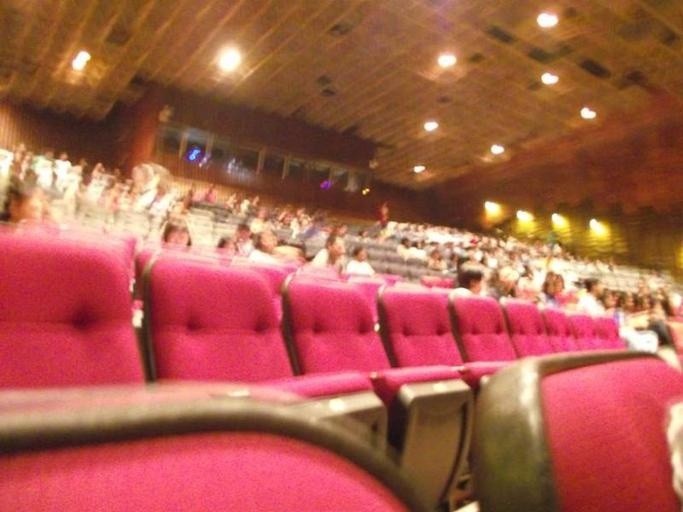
445 236 680 508
1 204 478 512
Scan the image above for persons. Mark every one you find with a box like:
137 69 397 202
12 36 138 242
121 156 379 323
377 202 682 351
2 146 124 235
